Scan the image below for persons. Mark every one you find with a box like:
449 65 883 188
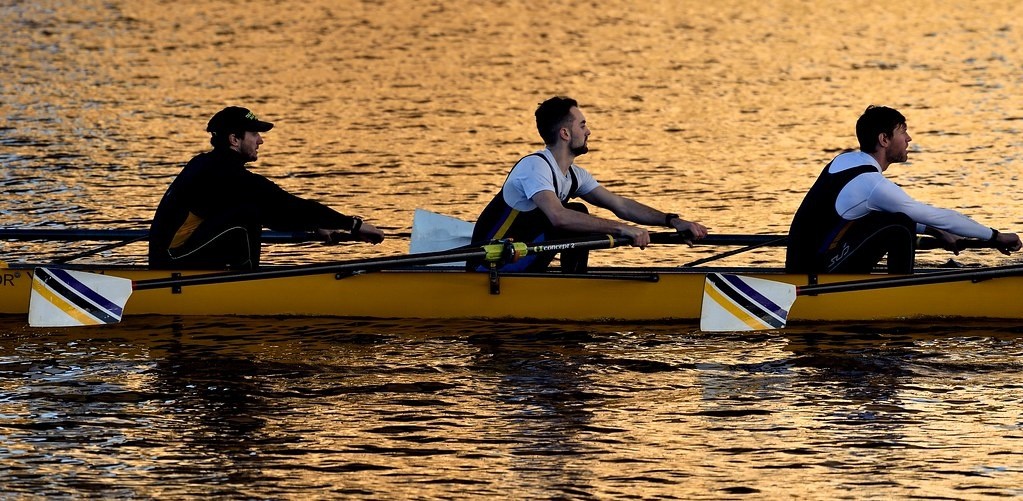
148 106 384 274
785 103 1023 276
466 96 708 276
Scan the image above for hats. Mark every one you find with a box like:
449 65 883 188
206 106 274 132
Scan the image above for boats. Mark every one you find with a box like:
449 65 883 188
0 222 1023 331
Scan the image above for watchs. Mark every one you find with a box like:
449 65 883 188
665 213 679 229
989 227 998 244
352 216 363 236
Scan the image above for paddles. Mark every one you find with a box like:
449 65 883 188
0 221 1022 333
